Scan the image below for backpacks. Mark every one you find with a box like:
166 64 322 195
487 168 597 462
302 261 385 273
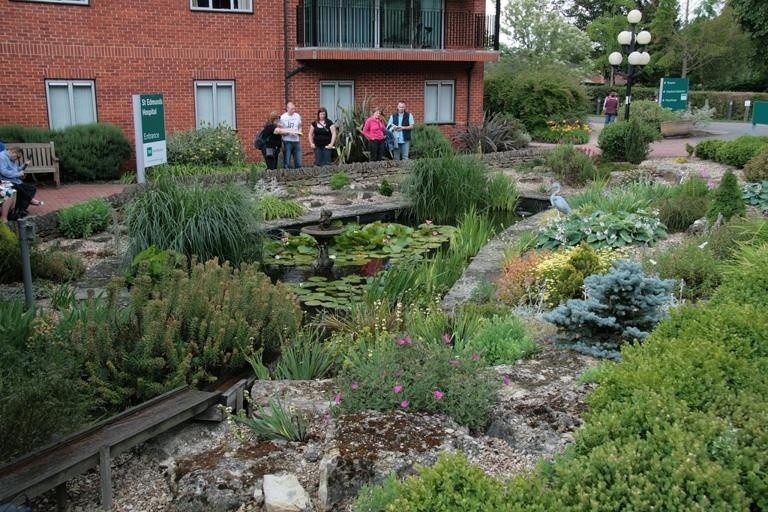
254 123 277 150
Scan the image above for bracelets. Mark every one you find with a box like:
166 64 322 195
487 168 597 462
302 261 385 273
286 130 290 134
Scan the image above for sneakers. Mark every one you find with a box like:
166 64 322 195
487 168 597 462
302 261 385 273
6 198 44 222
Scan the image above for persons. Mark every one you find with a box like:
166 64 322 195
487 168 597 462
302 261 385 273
0 145 37 219
361 105 387 163
258 109 296 170
602 93 618 128
13 146 44 206
277 100 303 168
603 92 619 122
384 99 415 161
0 178 19 224
307 106 337 166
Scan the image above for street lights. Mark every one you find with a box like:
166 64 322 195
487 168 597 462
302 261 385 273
606 8 654 122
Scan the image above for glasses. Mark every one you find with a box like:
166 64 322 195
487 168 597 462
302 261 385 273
14 151 22 160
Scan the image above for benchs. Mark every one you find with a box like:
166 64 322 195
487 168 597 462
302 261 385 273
4 140 61 190
